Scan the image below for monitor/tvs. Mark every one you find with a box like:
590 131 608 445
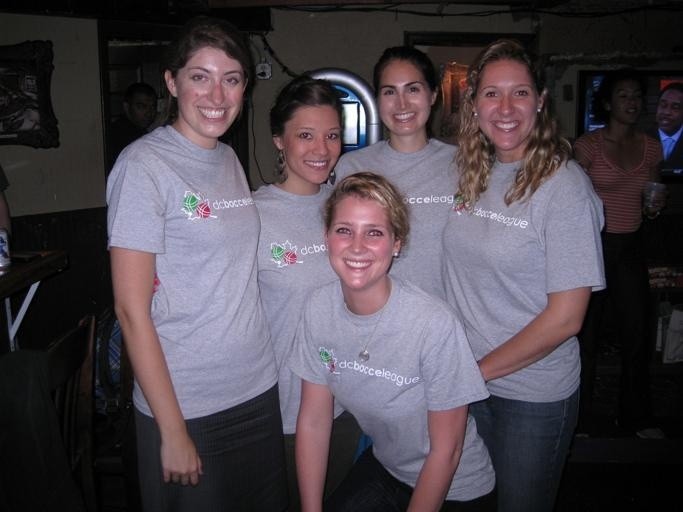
575 68 683 181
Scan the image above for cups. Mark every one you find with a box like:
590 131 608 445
644 181 665 213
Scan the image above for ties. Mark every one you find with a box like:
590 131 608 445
662 138 673 160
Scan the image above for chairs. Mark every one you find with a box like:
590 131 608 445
14 312 100 511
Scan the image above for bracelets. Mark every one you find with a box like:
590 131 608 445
642 206 661 220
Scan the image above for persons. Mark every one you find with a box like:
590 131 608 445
436 35 609 511
280 171 500 512
333 42 469 310
654 81 683 172
102 78 160 177
244 72 364 512
0 166 15 245
103 11 295 512
570 63 668 445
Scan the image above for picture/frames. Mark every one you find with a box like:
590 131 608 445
0 36 64 154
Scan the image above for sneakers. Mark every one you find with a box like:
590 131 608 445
636 426 663 441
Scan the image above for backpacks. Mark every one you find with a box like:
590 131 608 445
52 305 121 459
